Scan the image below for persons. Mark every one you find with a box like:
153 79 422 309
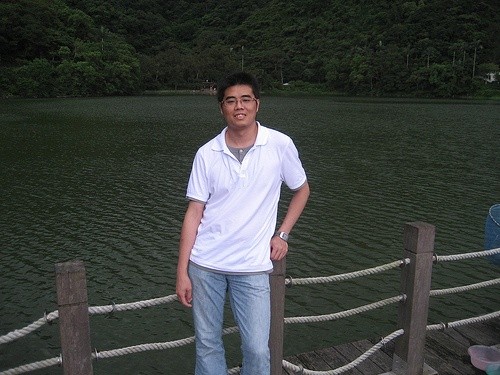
174 72 311 375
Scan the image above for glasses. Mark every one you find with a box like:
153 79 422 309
221 97 256 104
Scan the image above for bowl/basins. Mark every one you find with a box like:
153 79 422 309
467 345 500 371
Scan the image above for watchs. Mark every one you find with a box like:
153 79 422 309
274 231 288 243
